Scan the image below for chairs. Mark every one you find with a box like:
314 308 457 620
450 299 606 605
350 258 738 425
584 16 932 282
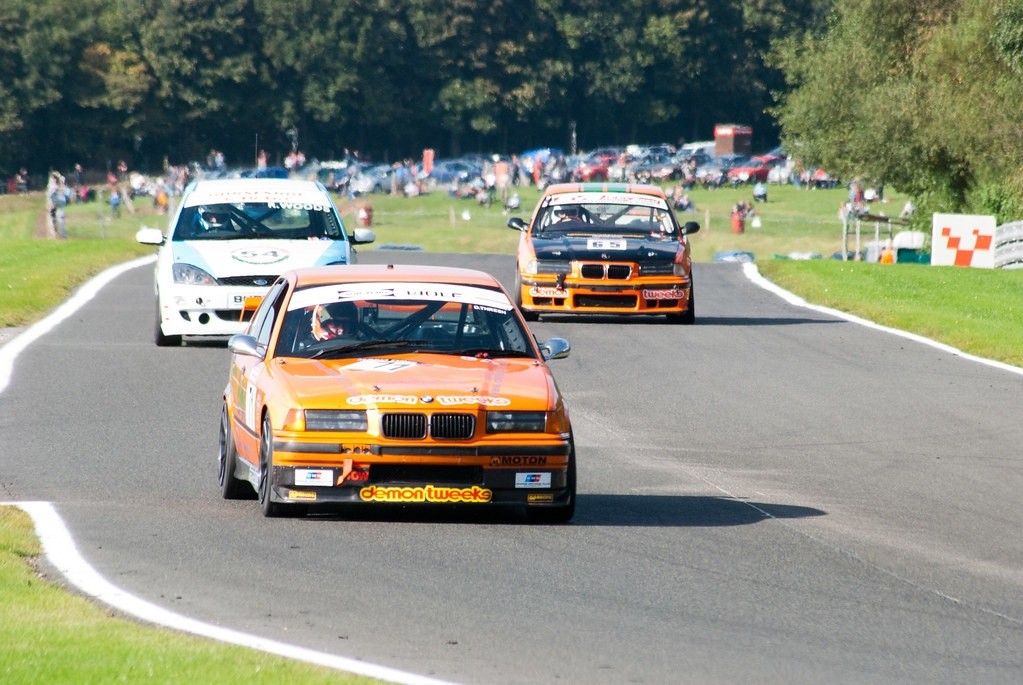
416 327 452 342
298 311 313 348
192 213 236 233
548 205 590 225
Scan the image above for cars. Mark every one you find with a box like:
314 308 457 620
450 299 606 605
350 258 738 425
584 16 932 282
136 176 377 347
218 139 885 201
507 182 701 325
217 263 578 527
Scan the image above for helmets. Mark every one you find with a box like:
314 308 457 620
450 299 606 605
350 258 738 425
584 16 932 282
549 205 580 224
198 205 231 231
312 302 357 342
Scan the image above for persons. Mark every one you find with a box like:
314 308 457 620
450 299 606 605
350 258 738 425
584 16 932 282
311 301 362 345
9 140 919 241
